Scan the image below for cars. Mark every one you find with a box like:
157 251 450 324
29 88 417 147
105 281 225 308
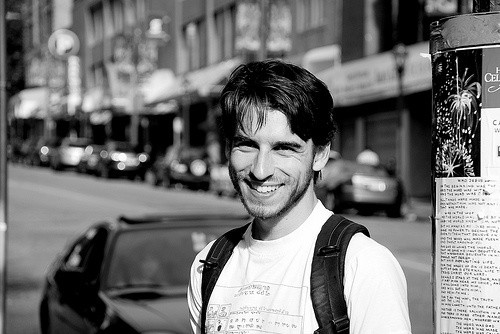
8 136 240 199
313 159 399 217
39 212 255 334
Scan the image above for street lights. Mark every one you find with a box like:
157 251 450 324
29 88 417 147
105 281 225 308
392 42 410 205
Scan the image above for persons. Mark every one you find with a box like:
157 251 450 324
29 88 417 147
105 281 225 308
187 60 412 334
